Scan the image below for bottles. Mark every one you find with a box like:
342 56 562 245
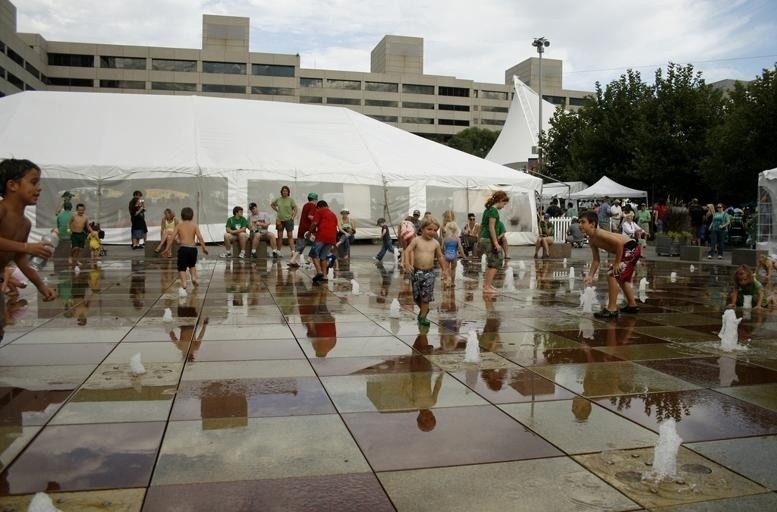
27 227 59 271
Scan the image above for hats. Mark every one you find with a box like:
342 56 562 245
247 202 258 211
307 191 319 201
375 218 386 227
338 208 350 216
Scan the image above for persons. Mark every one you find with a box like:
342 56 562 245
163 287 211 369
399 214 450 331
246 202 277 258
3 298 28 327
154 206 209 289
128 259 147 310
56 190 102 268
510 197 759 260
574 210 641 321
221 258 248 310
303 299 336 360
435 302 466 361
286 192 356 283
220 206 248 257
754 253 777 287
396 210 511 288
1 264 29 297
475 300 509 394
128 190 148 249
731 307 766 348
569 320 638 426
477 190 510 293
245 261 266 308
403 331 442 433
270 186 298 257
61 265 90 334
724 266 764 316
372 218 394 260
270 260 296 333
0 155 55 319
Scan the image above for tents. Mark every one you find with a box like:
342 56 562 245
566 176 648 209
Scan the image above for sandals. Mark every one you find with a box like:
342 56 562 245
592 307 618 320
417 308 431 327
619 304 641 314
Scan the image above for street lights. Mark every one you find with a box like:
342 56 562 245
532 34 552 177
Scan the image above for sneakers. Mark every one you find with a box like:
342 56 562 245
271 249 278 259
707 255 723 260
236 249 245 259
249 251 257 259
218 248 234 259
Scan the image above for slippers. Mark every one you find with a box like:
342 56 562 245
285 260 301 268
310 271 323 283
319 276 329 282
